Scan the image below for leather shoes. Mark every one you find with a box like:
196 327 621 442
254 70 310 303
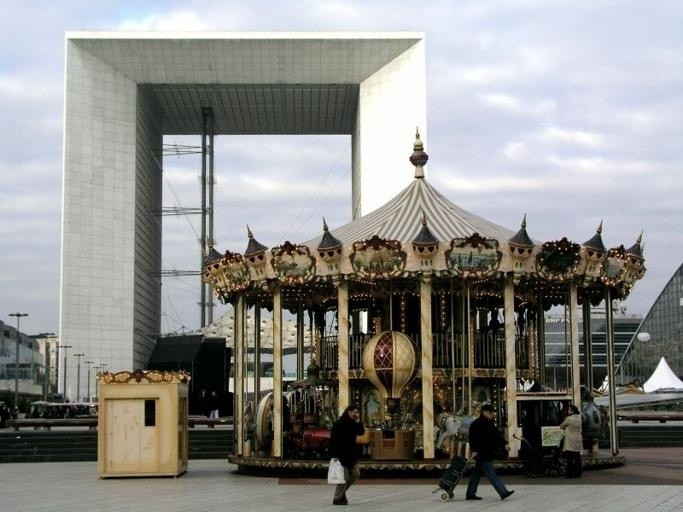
501 490 515 501
333 493 347 505
466 495 482 500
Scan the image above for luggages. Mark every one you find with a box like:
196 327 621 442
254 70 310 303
438 452 476 501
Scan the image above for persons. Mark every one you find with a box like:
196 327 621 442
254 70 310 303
466 404 515 501
209 391 219 419
559 405 583 478
329 404 364 505
0 401 98 429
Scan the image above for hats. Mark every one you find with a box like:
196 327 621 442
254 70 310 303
481 404 495 412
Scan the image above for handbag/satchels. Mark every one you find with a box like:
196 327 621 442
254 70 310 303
327 458 346 484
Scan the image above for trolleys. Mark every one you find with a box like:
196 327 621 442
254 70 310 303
432 455 473 502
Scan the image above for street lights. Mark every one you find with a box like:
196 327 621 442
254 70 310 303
93 363 108 402
74 353 85 403
9 312 29 410
85 361 93 402
59 345 73 403
